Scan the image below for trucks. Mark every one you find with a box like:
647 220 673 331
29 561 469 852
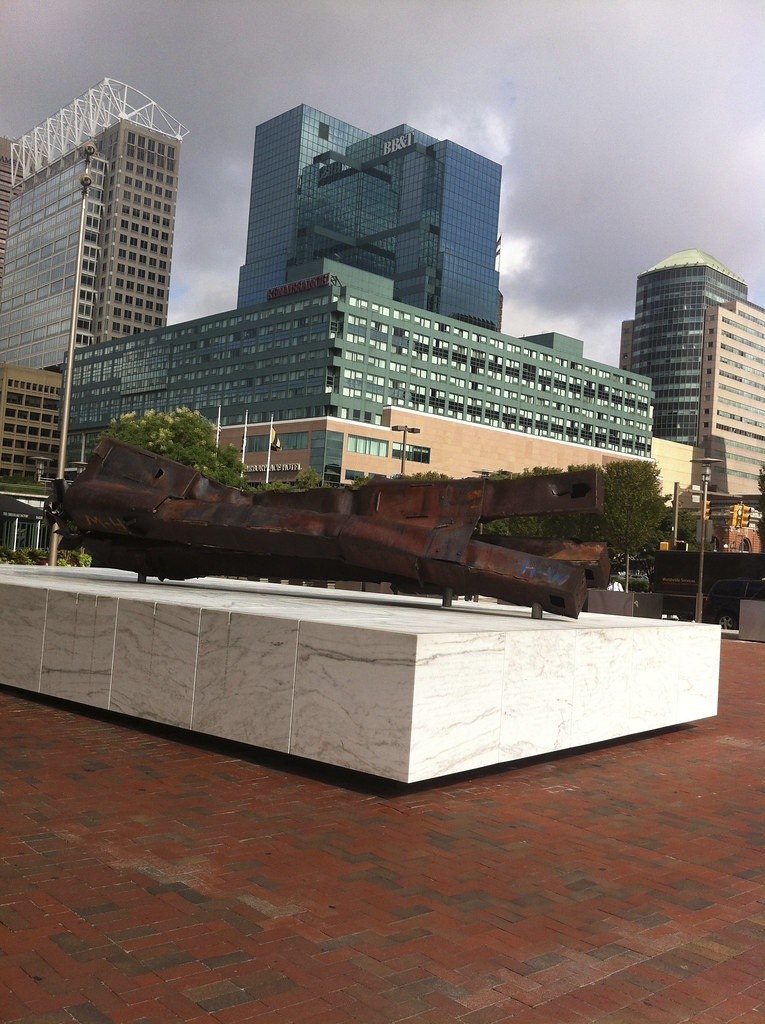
652 551 765 622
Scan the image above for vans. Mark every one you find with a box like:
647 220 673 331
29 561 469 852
702 578 765 630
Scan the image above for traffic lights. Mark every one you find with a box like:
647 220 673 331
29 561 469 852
727 500 752 528
705 500 712 521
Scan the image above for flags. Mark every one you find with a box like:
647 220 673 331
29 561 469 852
218 422 221 440
271 427 282 452
240 431 244 453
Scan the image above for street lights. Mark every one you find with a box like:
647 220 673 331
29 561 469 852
690 456 724 624
28 456 54 482
392 424 420 476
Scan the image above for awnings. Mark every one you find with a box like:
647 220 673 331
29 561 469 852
0 494 45 520
